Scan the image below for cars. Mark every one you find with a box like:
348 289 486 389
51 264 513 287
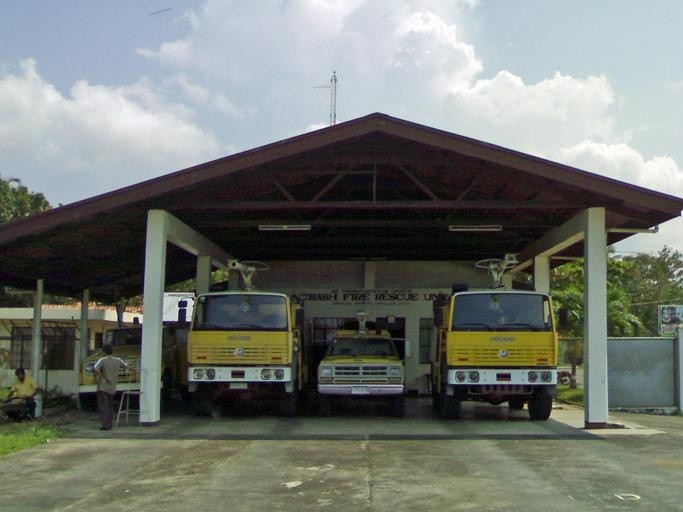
317 328 405 417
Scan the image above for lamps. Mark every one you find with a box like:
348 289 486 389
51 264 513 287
448 224 504 231
257 224 312 230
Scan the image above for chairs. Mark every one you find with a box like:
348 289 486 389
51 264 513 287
115 369 151 427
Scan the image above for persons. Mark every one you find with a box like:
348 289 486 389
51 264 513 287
6 368 40 420
93 345 139 431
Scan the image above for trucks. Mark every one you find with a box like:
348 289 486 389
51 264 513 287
77 326 177 409
178 291 305 416
432 286 559 420
